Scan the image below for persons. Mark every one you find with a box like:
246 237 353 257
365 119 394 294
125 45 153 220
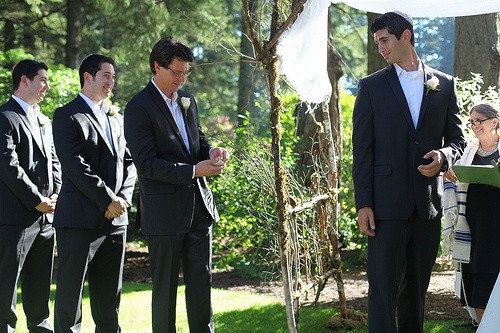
353 12 467 333
53 53 137 333
124 36 228 333
1 59 62 327
442 104 500 324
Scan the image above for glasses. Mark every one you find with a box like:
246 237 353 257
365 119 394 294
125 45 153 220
466 118 489 128
167 67 192 77
